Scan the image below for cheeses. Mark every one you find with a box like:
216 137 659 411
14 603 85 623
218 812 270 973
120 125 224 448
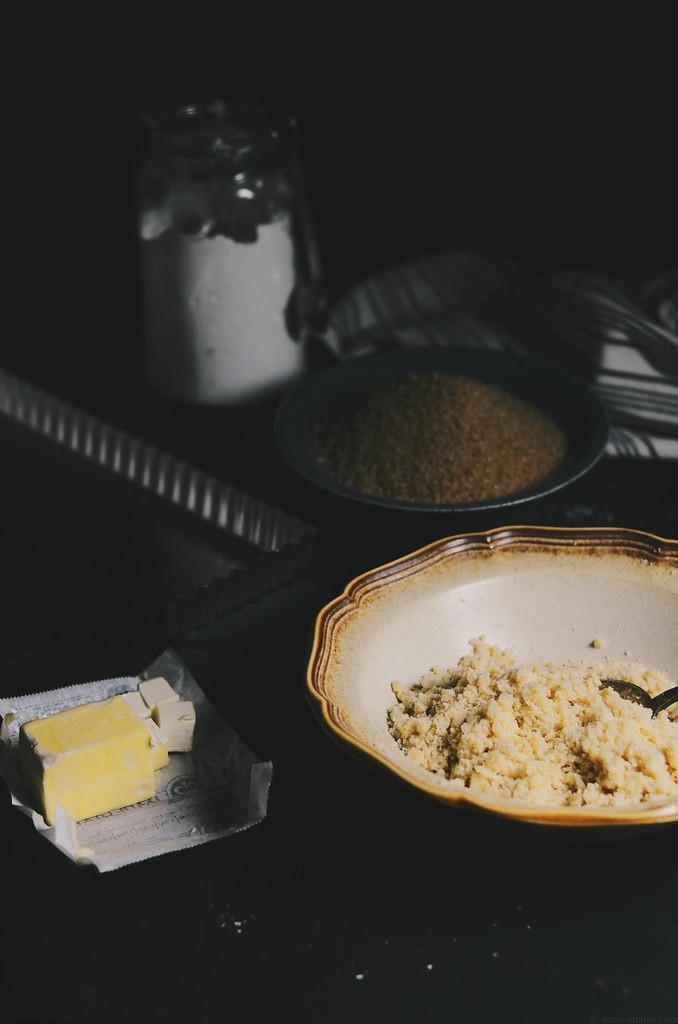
19 675 196 826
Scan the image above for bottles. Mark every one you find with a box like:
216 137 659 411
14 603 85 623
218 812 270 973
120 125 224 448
135 80 306 409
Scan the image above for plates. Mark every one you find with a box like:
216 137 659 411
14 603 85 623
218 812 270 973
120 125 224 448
268 348 612 511
306 526 677 839
